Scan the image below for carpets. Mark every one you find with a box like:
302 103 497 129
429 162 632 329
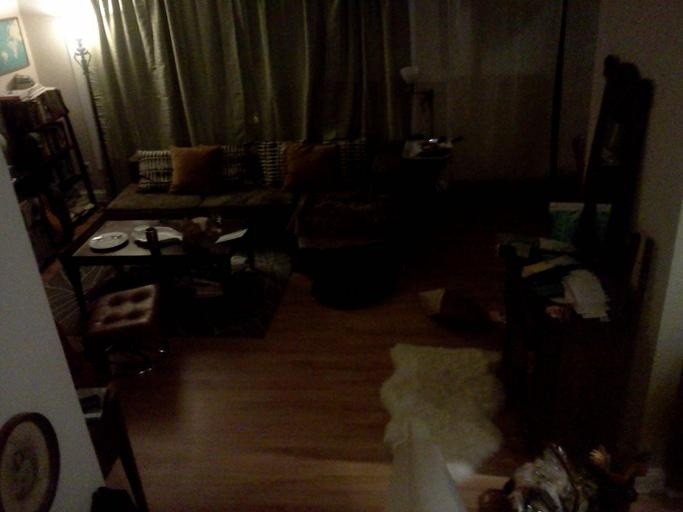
377 343 507 484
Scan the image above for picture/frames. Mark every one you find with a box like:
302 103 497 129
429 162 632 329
0 412 61 511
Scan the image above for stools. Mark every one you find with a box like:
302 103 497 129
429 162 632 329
85 284 173 372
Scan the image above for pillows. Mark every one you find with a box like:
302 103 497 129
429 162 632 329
136 139 370 195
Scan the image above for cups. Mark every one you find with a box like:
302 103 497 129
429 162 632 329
191 216 208 232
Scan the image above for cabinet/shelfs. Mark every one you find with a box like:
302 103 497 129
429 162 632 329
0 89 97 255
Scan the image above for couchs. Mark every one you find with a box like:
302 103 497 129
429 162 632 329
104 140 404 251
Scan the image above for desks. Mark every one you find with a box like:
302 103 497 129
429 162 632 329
495 242 639 457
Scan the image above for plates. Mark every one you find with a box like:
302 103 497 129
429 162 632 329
88 231 127 251
